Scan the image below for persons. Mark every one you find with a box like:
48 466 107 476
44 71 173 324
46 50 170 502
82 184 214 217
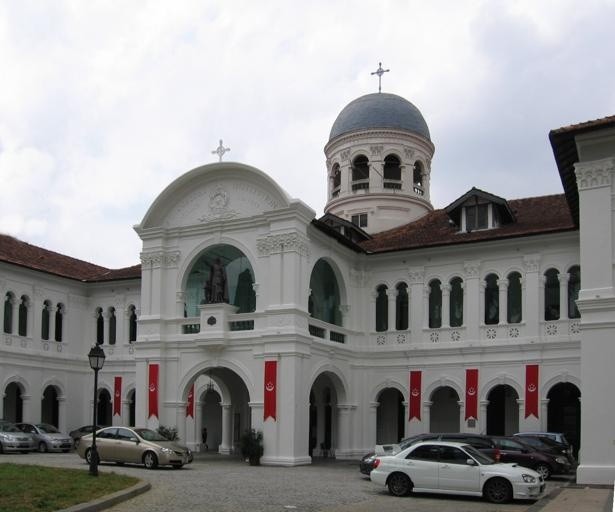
202 428 208 450
204 257 226 304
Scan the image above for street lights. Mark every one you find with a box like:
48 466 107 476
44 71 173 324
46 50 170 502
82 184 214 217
87 342 105 476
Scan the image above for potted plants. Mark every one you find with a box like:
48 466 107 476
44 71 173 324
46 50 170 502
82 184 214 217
240 427 264 465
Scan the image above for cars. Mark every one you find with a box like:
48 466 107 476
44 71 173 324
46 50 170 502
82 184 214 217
359 432 574 503
0 422 193 469
